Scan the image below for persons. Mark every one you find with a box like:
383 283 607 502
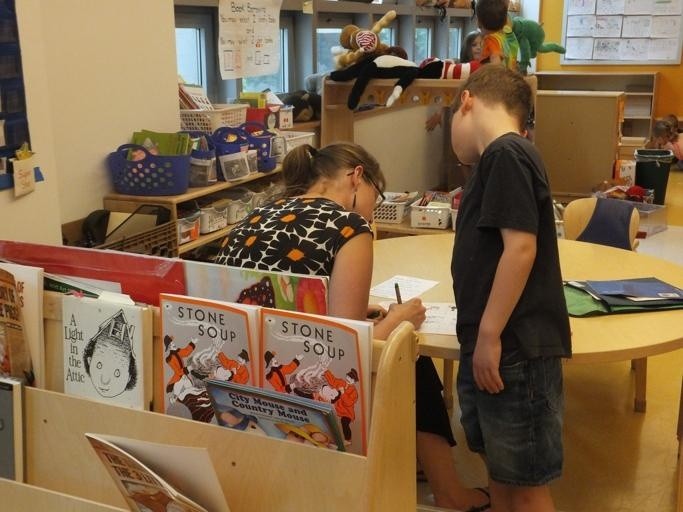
460 30 483 64
449 62 572 512
214 141 491 512
475 0 521 70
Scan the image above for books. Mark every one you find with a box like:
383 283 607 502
120 76 223 182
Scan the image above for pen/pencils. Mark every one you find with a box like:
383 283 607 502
395 283 402 304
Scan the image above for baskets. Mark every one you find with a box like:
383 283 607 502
176 175 299 246
372 192 410 224
410 198 457 231
180 104 248 133
108 131 315 196
61 214 178 268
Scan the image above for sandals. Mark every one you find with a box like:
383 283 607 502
467 486 491 512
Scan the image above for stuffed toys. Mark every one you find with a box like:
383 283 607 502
282 10 481 122
505 13 566 70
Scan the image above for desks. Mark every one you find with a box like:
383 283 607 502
366 232 683 440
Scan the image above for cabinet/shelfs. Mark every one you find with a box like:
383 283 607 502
2 290 417 512
534 70 659 139
102 163 285 253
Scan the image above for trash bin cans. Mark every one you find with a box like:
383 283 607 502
635 150 673 205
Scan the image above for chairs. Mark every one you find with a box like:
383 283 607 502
561 196 639 252
